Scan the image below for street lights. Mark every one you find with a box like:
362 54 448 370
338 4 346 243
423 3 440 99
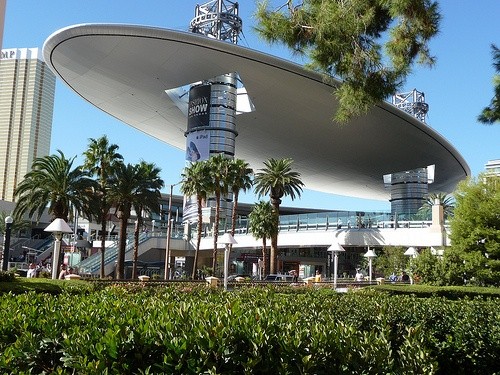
163 180 185 281
44 217 74 280
215 233 238 292
327 242 346 291
1 215 14 272
363 250 379 281
403 246 421 284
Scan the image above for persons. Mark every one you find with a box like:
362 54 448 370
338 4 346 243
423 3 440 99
315 216 419 284
77 224 168 245
10 249 73 281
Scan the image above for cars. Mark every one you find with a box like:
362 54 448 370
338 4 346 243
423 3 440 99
264 273 303 283
